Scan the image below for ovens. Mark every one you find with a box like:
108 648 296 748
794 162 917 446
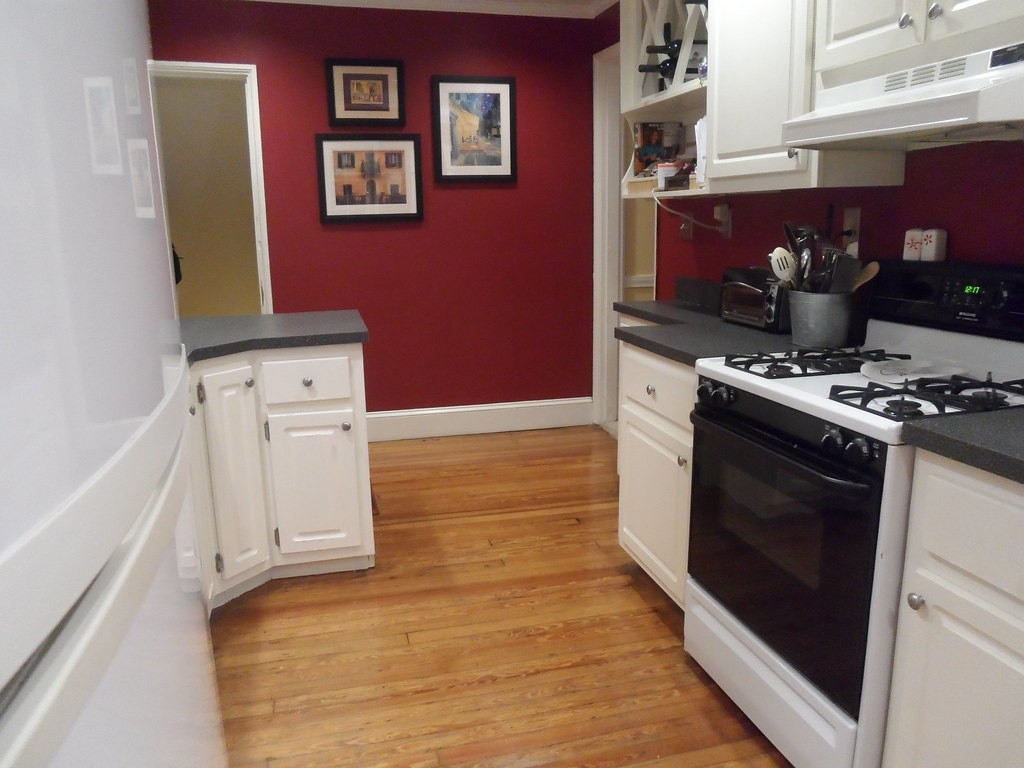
680 406 914 768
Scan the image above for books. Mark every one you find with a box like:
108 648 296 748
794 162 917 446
633 120 682 176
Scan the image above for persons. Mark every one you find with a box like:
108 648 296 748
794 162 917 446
639 128 665 167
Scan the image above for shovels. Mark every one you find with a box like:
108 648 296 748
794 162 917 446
852 261 879 292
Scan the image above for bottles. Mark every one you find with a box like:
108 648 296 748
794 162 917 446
638 39 681 79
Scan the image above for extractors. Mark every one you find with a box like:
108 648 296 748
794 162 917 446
779 39 1023 154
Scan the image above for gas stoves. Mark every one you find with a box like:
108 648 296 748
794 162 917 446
695 344 1023 445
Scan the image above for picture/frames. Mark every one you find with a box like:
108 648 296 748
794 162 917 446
316 131 425 222
325 57 407 129
430 73 519 184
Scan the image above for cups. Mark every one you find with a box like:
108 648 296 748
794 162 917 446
787 287 855 349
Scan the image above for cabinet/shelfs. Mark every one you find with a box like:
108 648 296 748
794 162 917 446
879 444 1024 768
612 343 700 610
616 0 1024 199
185 343 378 618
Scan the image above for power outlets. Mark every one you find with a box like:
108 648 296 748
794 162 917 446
843 206 859 256
723 211 732 241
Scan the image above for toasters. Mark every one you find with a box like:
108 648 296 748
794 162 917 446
718 265 790 333
